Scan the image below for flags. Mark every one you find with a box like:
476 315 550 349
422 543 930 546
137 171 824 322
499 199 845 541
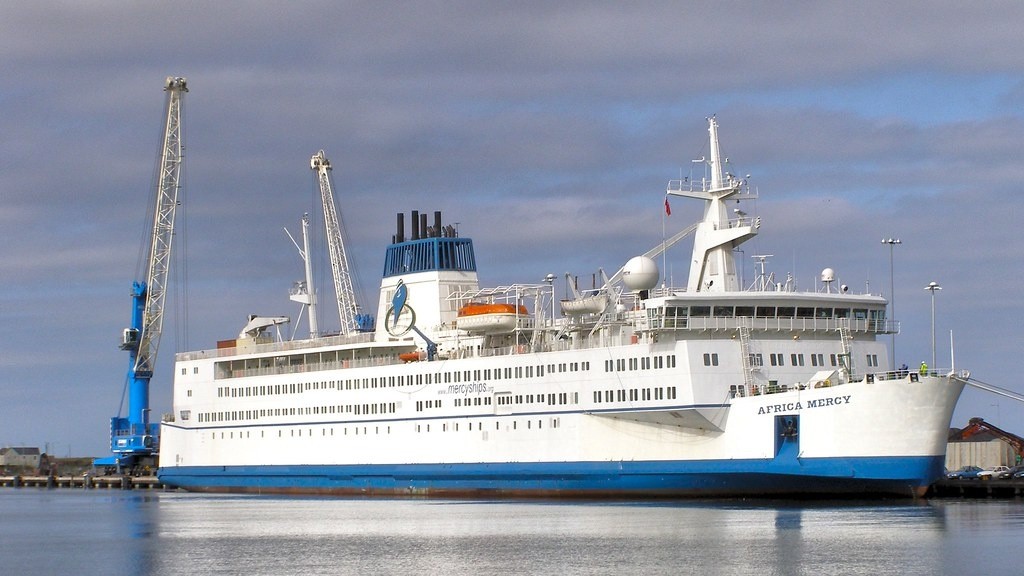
665 196 671 216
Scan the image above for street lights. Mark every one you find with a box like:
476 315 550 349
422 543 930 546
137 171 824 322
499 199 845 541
924 280 942 371
879 239 901 369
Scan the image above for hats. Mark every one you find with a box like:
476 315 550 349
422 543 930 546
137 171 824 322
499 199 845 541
921 361 925 363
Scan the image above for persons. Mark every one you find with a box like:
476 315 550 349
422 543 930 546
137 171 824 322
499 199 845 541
896 364 908 379
920 361 928 377
751 385 760 396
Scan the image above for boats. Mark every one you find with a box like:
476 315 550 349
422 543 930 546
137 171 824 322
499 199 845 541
154 111 974 506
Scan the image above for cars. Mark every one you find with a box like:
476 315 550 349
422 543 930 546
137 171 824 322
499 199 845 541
1014 469 1024 480
977 466 1009 480
943 467 949 478
999 464 1024 481
947 466 984 480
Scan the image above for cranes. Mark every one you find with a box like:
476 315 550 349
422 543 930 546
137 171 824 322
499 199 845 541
94 76 190 476
